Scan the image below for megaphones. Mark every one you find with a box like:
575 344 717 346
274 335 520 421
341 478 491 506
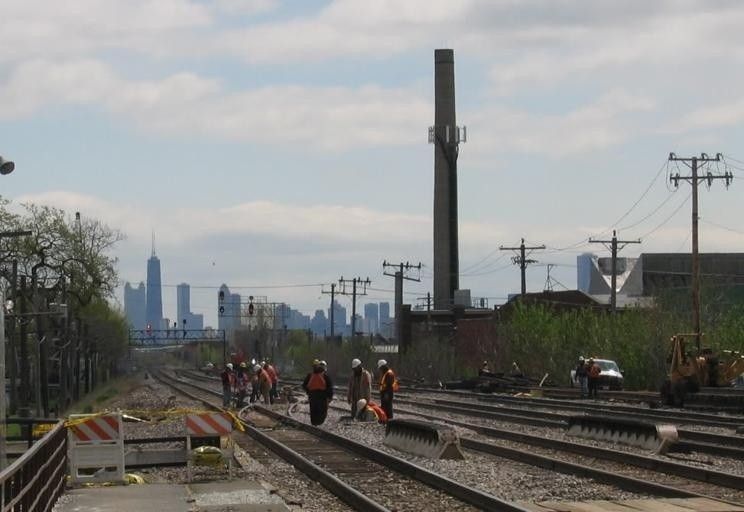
0 155 15 175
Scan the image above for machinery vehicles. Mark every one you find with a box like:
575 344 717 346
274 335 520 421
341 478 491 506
662 333 744 395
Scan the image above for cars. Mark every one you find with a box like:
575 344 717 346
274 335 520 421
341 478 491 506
570 358 624 391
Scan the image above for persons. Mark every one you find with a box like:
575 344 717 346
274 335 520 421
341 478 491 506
509 362 523 377
346 357 372 418
353 399 386 422
585 357 601 397
478 361 491 372
375 359 398 424
303 358 327 427
574 355 587 399
219 357 275 406
305 361 332 416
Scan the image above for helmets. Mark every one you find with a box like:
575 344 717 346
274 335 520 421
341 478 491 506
377 359 387 368
317 360 327 371
356 399 366 412
226 361 266 373
312 359 319 367
578 355 585 362
351 358 361 369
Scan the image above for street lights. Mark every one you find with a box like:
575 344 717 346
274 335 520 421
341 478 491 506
5 258 17 414
